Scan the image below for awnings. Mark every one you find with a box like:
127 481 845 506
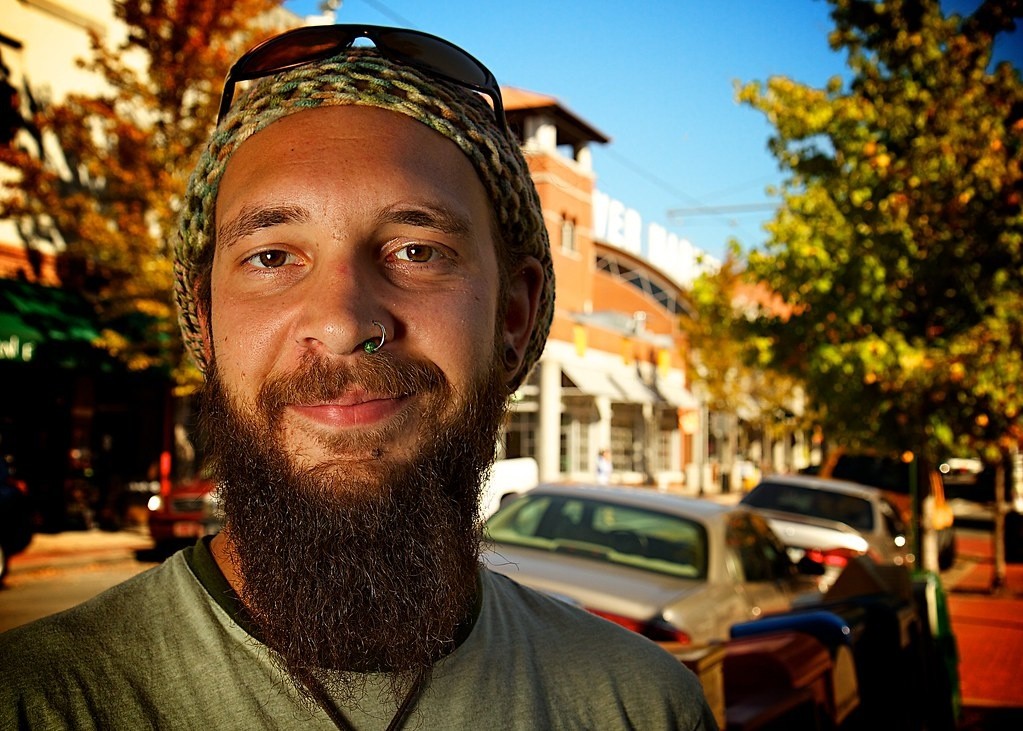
0 280 175 378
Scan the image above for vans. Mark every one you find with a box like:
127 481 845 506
822 446 957 569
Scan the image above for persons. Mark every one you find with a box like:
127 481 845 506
0 24 717 731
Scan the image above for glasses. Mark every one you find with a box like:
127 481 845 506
215 25 510 138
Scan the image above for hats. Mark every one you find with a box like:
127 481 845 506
175 48 554 392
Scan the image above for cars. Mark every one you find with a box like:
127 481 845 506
735 474 920 593
148 443 239 556
934 447 987 498
474 480 827 663
